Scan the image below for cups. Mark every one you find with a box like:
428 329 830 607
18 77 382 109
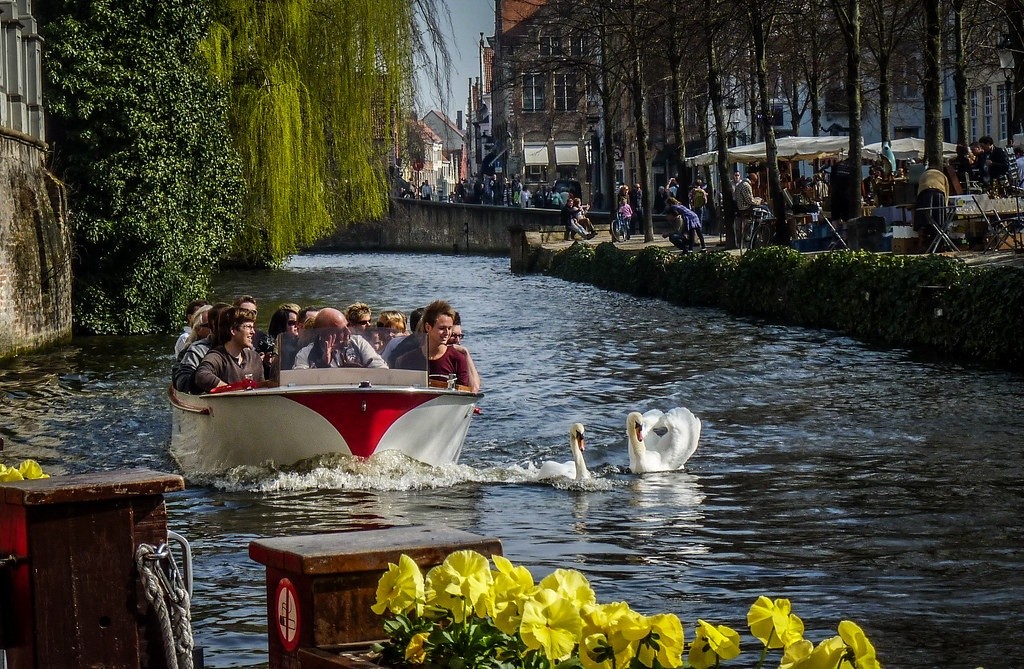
957 197 1021 213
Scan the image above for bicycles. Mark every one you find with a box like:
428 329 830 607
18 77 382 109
745 205 773 250
608 207 629 241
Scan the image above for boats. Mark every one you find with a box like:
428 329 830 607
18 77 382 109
168 328 485 492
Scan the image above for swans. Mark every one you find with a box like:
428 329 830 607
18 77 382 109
626 407 702 474
537 423 592 481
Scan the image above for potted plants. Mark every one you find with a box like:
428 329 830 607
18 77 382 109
949 223 961 239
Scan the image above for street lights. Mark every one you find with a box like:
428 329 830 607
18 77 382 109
999 48 1017 147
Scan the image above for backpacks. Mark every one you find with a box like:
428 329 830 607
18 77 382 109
693 189 705 208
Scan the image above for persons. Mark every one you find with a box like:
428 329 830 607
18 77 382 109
172 296 481 394
404 136 1024 255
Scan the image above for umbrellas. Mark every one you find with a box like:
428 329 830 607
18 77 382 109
727 137 862 182
861 137 971 167
685 146 761 245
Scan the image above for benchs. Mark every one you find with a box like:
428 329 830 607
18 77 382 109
539 224 611 241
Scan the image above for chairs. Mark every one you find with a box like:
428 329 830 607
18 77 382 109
965 171 982 195
972 194 1024 253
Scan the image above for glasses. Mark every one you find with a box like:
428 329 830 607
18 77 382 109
356 319 372 324
237 325 255 329
251 310 258 316
377 322 385 328
199 323 209 328
451 333 465 340
287 320 298 326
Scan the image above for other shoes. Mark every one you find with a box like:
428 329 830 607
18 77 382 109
586 234 594 240
592 230 599 235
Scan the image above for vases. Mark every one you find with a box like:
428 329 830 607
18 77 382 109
298 639 401 669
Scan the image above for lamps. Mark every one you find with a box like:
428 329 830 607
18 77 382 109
480 130 489 139
587 126 595 133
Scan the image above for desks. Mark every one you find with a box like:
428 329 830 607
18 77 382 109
785 207 821 226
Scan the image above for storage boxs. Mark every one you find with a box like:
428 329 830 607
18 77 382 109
789 235 847 253
890 226 919 254
984 232 1024 250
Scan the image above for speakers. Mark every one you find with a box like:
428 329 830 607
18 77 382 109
830 165 858 221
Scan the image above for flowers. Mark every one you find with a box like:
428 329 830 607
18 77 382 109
368 547 883 669
0 460 50 483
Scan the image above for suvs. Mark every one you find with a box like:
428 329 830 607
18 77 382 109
553 178 583 206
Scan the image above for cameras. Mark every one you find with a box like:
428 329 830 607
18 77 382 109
255 334 275 357
